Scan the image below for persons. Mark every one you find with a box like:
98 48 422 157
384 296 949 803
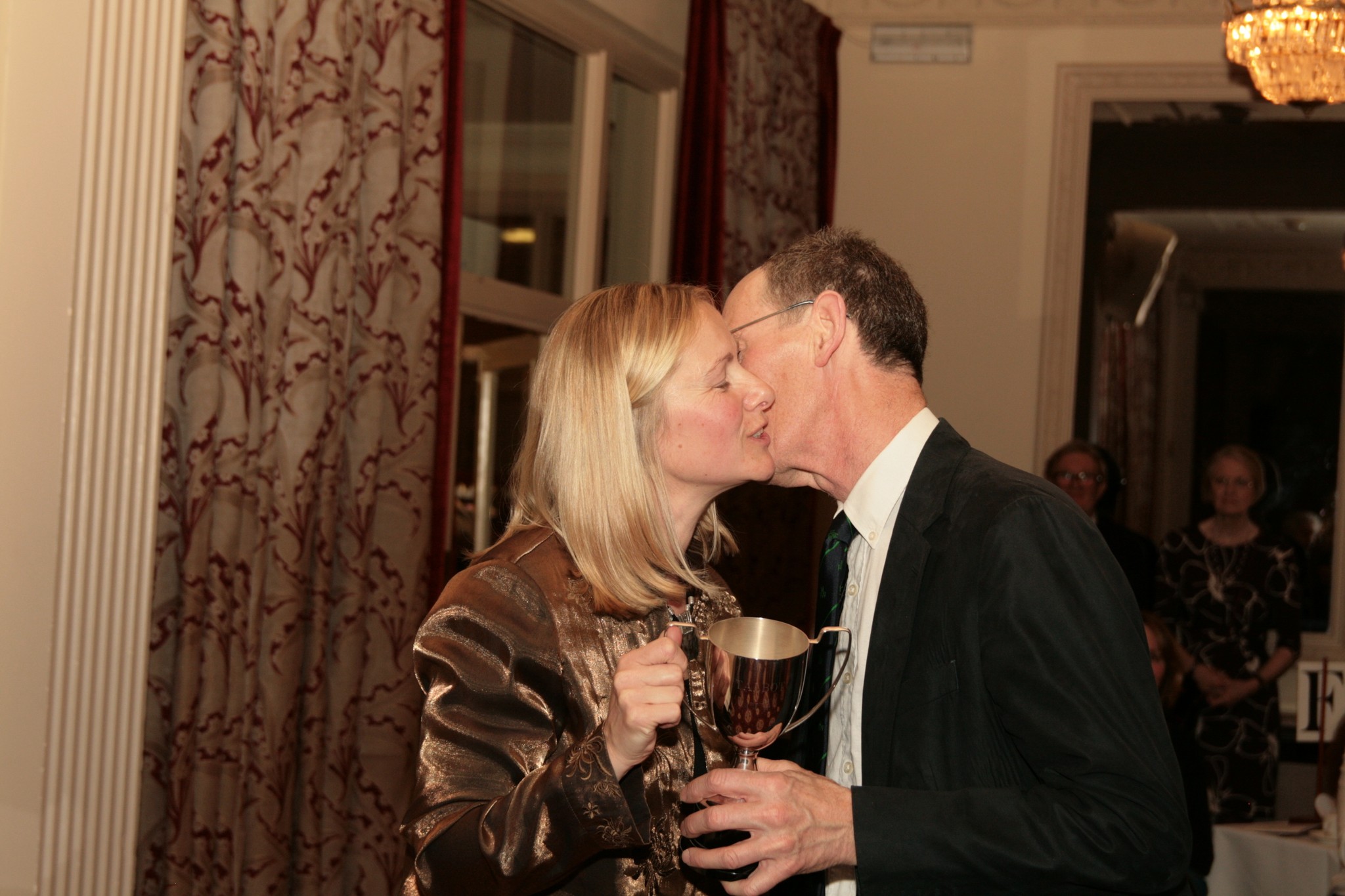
405 283 777 896
1158 444 1302 824
1142 615 1215 896
1043 438 1162 618
681 226 1196 896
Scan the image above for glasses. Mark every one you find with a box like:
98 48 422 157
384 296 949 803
1055 470 1098 488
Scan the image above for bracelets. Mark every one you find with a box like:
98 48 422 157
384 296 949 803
1252 672 1265 688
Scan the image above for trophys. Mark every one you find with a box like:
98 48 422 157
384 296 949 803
663 623 853 881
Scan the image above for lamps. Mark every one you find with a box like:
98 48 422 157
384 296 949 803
1222 0 1345 119
